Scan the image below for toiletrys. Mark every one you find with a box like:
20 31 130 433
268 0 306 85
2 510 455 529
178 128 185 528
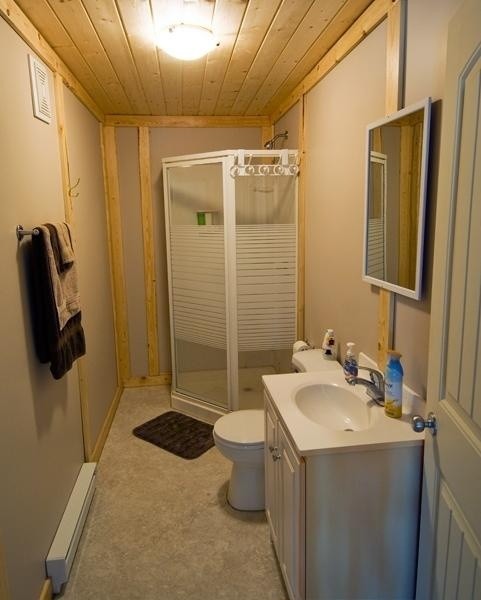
341 340 359 384
379 344 406 419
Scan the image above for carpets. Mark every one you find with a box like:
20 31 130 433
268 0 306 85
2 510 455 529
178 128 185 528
132 408 215 460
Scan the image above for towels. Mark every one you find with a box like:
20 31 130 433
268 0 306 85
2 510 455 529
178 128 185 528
44 222 75 264
36 226 81 331
25 228 86 381
42 224 73 273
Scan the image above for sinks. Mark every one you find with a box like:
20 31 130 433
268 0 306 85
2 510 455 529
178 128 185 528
294 382 370 433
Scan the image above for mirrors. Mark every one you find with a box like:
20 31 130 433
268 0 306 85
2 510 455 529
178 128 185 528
362 96 432 300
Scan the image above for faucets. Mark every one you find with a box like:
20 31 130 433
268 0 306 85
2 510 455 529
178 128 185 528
345 363 385 408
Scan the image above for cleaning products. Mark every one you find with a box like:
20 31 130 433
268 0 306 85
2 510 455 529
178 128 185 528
321 328 339 361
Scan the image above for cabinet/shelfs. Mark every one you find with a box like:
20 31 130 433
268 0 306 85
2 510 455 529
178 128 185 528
264 390 425 600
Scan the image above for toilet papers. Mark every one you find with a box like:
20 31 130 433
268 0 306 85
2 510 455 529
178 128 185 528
291 340 309 354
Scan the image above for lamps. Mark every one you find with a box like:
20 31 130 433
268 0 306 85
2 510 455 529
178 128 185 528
156 27 219 62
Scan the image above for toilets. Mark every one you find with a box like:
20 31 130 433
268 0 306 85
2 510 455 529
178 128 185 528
211 347 342 513
